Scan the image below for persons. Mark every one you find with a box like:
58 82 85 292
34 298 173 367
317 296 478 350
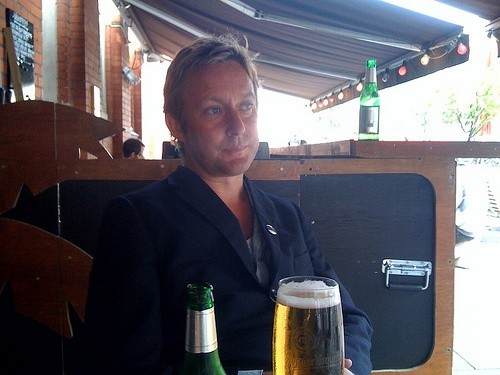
123 138 145 159
83 34 374 375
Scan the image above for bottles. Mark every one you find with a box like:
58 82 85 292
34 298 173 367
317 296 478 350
180 283 227 375
358 59 380 141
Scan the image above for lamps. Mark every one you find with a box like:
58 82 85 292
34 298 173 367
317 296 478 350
312 38 468 109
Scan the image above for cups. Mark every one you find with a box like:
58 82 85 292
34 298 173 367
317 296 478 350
272 276 344 375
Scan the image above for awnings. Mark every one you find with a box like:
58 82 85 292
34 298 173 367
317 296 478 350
434 0 500 57
112 0 470 113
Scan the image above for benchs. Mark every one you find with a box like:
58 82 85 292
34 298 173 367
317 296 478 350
0 159 455 374
